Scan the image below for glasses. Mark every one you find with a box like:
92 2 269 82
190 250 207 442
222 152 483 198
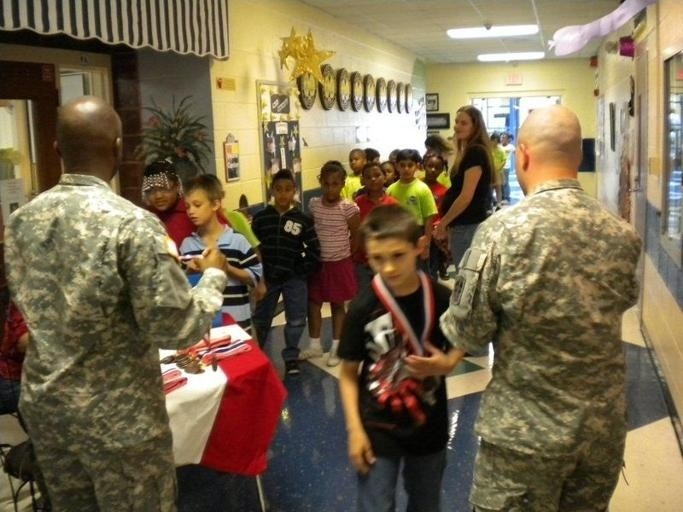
142 179 177 194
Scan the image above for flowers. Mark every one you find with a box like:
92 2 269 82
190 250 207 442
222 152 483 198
129 94 214 177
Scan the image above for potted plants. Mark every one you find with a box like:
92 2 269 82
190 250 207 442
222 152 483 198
0 147 24 180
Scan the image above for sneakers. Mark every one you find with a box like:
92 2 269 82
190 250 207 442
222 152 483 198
437 264 451 280
296 343 325 363
325 342 344 367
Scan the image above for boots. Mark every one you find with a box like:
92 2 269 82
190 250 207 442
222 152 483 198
280 346 303 375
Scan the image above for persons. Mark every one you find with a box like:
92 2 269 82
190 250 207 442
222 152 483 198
438 107 644 511
2 93 228 512
0 299 31 415
336 205 466 512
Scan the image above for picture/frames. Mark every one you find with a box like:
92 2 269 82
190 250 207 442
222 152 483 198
425 113 451 131
296 59 413 118
425 92 440 112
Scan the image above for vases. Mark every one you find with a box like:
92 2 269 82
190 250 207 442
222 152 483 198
172 158 198 185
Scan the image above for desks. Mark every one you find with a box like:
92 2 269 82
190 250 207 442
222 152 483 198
157 309 287 511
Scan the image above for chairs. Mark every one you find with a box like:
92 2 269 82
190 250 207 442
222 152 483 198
0 403 52 511
